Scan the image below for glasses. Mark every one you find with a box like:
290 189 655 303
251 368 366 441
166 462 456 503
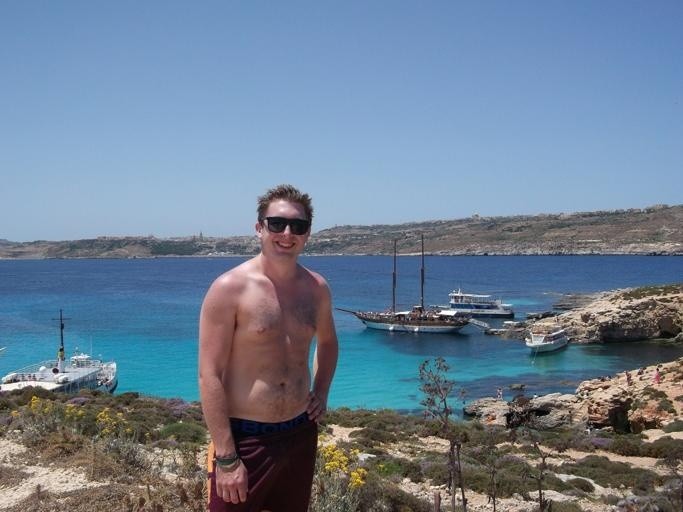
259 216 310 235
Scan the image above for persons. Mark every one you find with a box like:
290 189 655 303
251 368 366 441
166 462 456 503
194 184 340 512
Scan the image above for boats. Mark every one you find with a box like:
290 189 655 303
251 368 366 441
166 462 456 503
335 230 516 336
522 322 570 354
1 306 119 401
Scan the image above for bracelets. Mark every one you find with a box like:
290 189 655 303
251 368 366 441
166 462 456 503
211 451 241 472
215 455 238 467
